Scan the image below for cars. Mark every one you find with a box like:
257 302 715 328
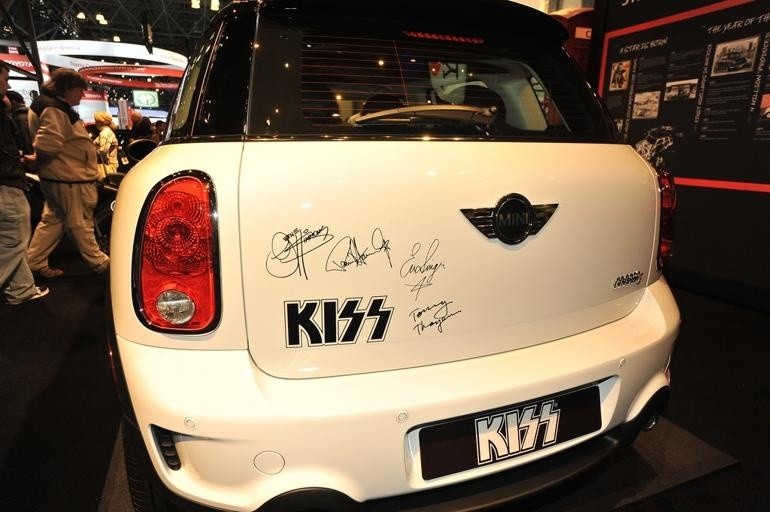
714 51 752 72
100 1 682 510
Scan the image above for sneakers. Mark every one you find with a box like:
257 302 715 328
7 286 50 305
37 265 64 279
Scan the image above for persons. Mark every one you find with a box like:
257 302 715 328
150 120 165 142
0 60 110 307
126 112 152 142
93 110 118 176
